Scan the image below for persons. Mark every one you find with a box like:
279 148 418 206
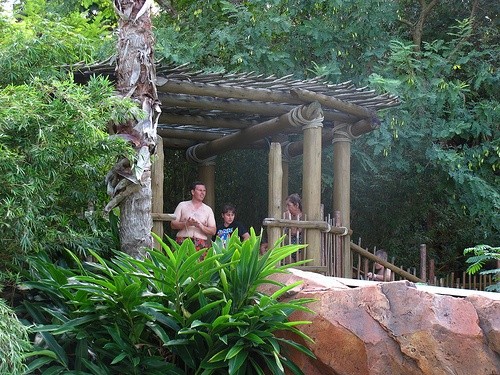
259 193 305 267
211 202 251 243
170 180 217 262
365 249 395 282
